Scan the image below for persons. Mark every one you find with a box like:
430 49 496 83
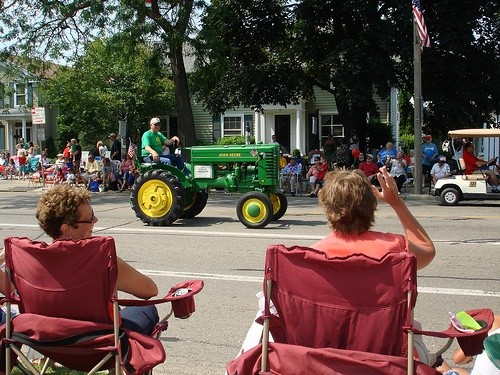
102 159 117 191
119 153 136 191
279 142 414 197
175 144 186 163
324 135 339 171
348 129 360 169
476 155 489 171
336 138 348 170
462 141 500 193
452 313 500 375
0 246 21 326
422 135 438 185
309 165 435 364
162 144 170 155
35 183 158 375
108 133 121 163
141 118 180 166
0 138 86 186
269 135 282 150
430 155 461 199
250 140 255 144
96 141 107 159
421 353 470 375
82 155 102 191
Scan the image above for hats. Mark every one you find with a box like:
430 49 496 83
150 118 160 124
367 153 374 158
57 154 63 157
438 155 445 161
424 135 432 139
108 133 116 137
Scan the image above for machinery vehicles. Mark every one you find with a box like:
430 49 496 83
127 122 288 230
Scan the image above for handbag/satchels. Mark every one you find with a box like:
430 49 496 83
98 184 105 192
89 182 100 191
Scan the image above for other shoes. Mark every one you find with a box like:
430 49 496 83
118 187 124 192
124 188 128 191
492 189 500 192
309 193 317 197
291 192 295 197
398 192 401 195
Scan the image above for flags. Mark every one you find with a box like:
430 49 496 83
128 136 135 158
412 0 431 55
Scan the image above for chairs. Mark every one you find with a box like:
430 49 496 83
459 158 489 180
226 244 495 375
0 237 204 375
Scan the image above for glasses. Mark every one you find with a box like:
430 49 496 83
469 145 474 148
70 212 94 224
153 123 160 126
